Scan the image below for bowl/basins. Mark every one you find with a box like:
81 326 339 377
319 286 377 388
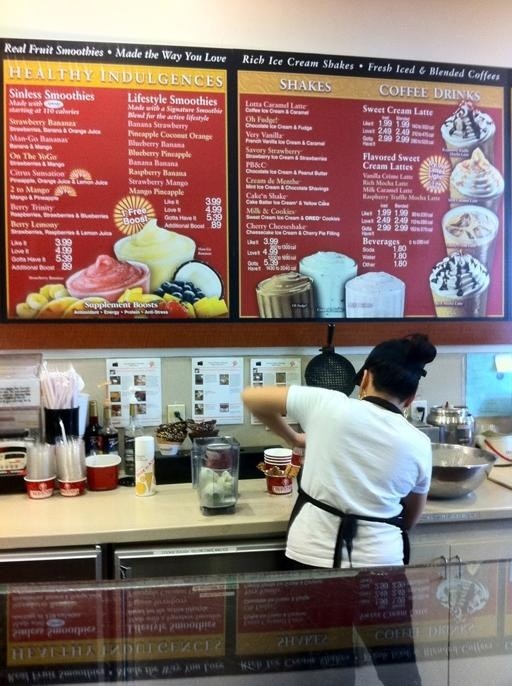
427 443 497 497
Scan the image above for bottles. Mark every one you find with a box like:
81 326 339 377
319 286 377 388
103 406 119 452
84 399 104 451
124 402 142 475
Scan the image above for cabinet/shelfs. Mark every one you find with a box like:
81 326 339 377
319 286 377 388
356 521 511 686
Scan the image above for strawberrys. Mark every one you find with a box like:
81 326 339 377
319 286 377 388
144 300 166 319
166 300 188 319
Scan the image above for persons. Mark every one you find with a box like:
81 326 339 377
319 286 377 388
240 333 437 686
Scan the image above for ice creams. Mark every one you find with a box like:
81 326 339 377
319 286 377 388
440 100 496 172
113 218 196 292
448 148 505 211
345 271 405 318
255 272 316 318
299 251 358 319
441 205 500 266
66 254 150 303
430 250 489 319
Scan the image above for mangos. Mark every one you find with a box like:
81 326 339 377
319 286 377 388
37 297 80 320
63 297 111 319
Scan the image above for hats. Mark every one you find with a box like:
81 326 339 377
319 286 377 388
353 341 427 386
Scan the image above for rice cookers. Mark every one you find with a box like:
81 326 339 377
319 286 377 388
426 402 476 447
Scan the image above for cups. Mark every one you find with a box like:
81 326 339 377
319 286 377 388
55 476 89 497
67 262 149 301
114 232 196 292
264 448 296 495
25 476 57 498
45 407 80 444
430 113 504 317
85 453 123 488
255 250 406 318
